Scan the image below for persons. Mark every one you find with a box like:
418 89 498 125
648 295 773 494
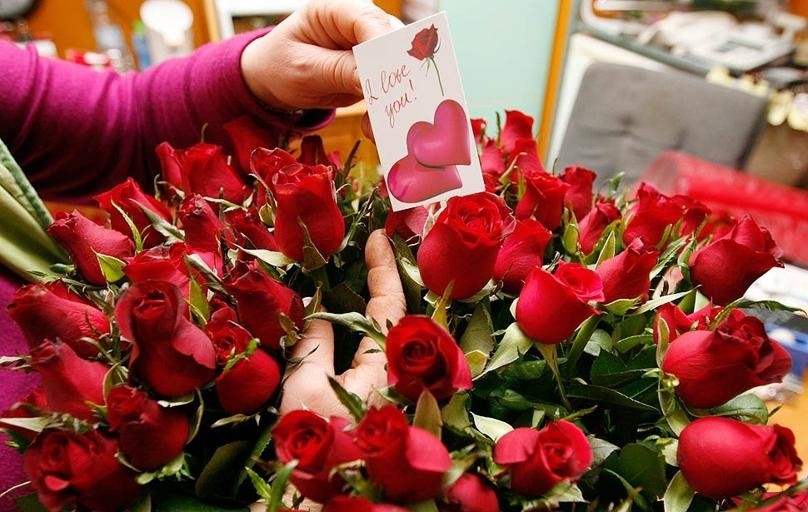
0 1 409 512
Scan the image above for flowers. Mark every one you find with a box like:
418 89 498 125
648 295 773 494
0 109 807 512
